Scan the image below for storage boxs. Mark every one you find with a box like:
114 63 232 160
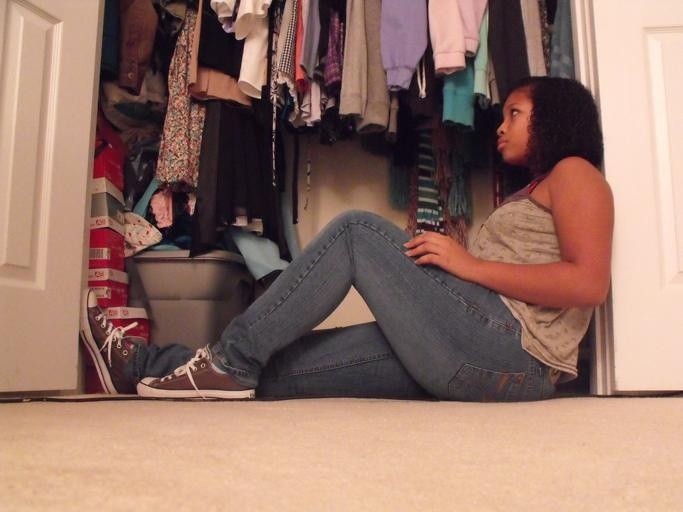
88 136 148 392
132 251 258 361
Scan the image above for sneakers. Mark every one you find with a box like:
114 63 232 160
79 287 140 397
134 341 257 401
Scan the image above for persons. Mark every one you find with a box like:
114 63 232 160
79 76 617 401
